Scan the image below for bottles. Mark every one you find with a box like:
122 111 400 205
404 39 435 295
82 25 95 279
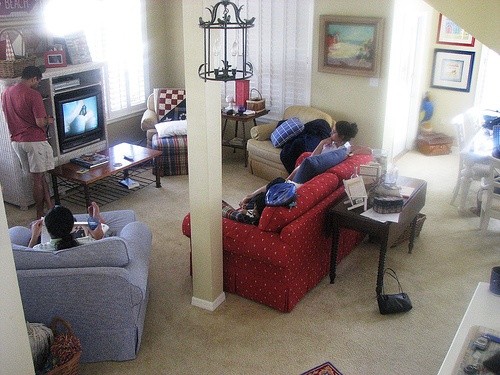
390 163 398 184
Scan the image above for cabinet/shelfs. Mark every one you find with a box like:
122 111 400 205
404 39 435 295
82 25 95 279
0 60 112 210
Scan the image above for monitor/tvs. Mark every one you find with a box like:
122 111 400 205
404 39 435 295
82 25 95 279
54 84 103 154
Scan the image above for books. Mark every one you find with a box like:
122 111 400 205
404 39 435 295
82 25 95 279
119 178 139 189
70 157 109 168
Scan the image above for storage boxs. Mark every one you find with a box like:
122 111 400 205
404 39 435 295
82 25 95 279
246 98 266 110
63 29 92 65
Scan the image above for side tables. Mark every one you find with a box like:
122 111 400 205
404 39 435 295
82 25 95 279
221 108 270 168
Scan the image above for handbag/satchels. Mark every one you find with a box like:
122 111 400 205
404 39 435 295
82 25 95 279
376 267 413 315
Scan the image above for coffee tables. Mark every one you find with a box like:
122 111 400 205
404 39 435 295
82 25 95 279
50 140 167 212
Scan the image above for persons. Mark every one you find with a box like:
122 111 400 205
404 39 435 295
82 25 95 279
287 121 358 181
27 202 103 250
1 66 55 219
239 177 286 226
79 105 87 116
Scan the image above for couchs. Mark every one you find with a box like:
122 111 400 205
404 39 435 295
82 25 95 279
10 205 157 363
142 84 189 177
179 144 378 317
247 102 339 183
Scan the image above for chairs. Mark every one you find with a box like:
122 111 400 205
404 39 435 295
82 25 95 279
450 113 483 208
478 153 500 235
466 108 483 136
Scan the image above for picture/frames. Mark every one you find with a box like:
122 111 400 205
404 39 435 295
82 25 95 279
317 14 386 79
435 12 475 47
429 48 475 93
45 50 65 68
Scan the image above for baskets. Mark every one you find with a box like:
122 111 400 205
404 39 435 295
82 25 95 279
36 317 83 375
418 132 454 155
1 28 36 77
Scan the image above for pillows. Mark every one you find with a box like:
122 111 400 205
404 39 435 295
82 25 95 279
269 116 306 148
291 146 348 182
155 120 186 139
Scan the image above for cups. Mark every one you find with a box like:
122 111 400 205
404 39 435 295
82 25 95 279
88 217 99 230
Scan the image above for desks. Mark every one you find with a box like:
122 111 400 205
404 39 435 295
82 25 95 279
454 124 500 216
325 176 431 300
434 281 500 375
